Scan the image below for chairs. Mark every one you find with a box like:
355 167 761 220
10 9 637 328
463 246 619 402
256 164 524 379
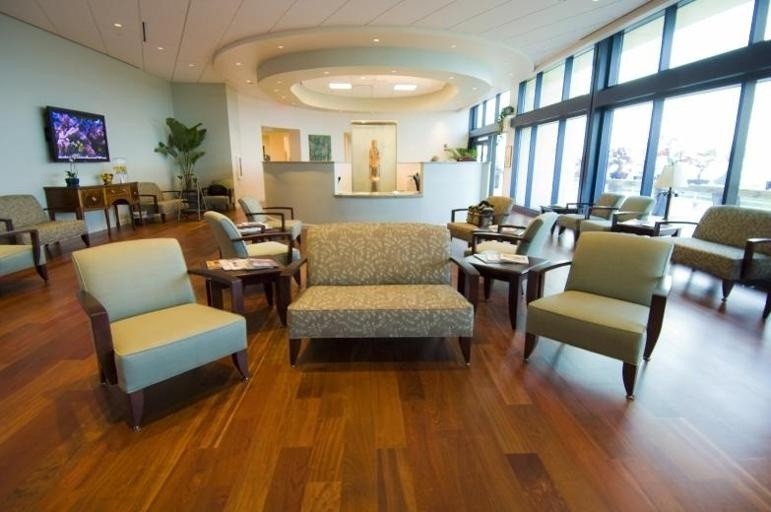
1 194 91 259
200 176 233 213
131 182 192 224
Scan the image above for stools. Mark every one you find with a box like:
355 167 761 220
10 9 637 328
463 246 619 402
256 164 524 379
1 218 52 281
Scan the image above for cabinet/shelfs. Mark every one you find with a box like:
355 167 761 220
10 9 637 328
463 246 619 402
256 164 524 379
41 181 143 247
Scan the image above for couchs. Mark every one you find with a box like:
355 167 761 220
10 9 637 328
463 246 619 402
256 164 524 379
283 222 476 370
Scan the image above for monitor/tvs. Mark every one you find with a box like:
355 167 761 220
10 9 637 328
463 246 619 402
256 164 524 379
43 105 110 163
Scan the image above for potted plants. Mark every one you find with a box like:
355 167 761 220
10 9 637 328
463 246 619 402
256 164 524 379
60 136 86 187
